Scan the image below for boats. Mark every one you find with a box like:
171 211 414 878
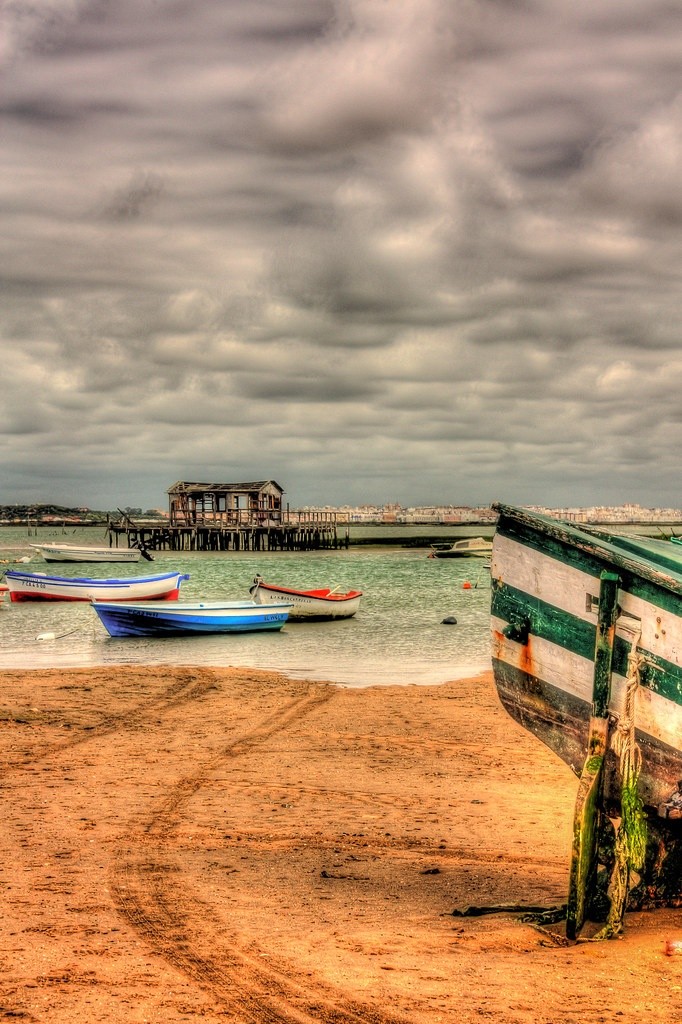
430 538 492 558
88 594 295 637
28 541 156 563
249 573 363 620
2 568 191 602
488 503 681 943
